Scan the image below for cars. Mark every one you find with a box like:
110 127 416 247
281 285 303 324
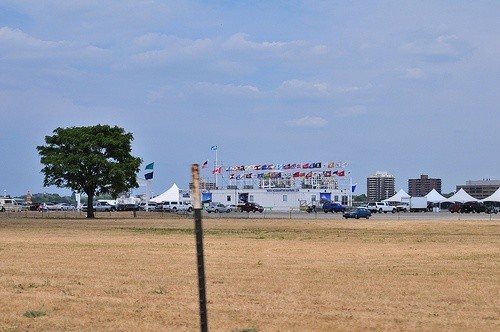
447 203 492 214
0 199 22 212
205 203 242 214
39 200 162 213
343 208 372 219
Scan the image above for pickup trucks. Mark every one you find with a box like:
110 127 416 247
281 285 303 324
237 202 264 213
367 201 397 214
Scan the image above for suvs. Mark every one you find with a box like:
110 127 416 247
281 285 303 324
322 202 347 213
161 200 193 213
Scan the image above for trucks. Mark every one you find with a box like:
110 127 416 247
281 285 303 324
396 196 427 212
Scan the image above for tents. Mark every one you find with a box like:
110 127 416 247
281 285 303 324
441 188 483 203
381 189 411 202
150 183 179 202
480 187 500 202
424 188 455 203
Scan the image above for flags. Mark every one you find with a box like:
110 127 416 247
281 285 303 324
226 161 351 180
212 167 221 174
145 162 154 170
211 145 217 150
202 161 208 168
144 171 153 180
